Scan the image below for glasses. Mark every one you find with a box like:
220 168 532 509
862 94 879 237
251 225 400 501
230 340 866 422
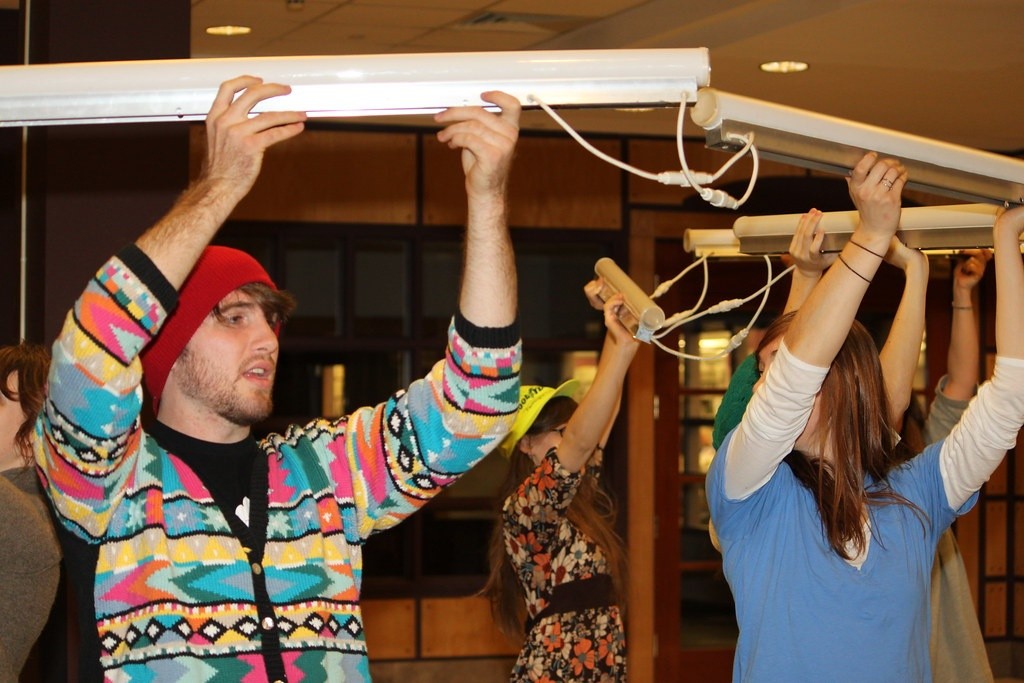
550 426 566 437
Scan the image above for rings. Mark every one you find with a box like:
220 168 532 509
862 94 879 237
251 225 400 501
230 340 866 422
883 178 893 185
880 182 892 189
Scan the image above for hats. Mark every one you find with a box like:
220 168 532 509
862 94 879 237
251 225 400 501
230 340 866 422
495 377 581 461
137 244 281 415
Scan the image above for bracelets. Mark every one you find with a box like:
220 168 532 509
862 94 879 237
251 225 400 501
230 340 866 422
837 252 872 284
947 300 974 311
849 238 886 260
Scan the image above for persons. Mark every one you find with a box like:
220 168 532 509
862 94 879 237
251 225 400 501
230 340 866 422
708 207 996 683
472 279 642 683
0 342 63 683
703 150 1024 683
28 72 523 683
